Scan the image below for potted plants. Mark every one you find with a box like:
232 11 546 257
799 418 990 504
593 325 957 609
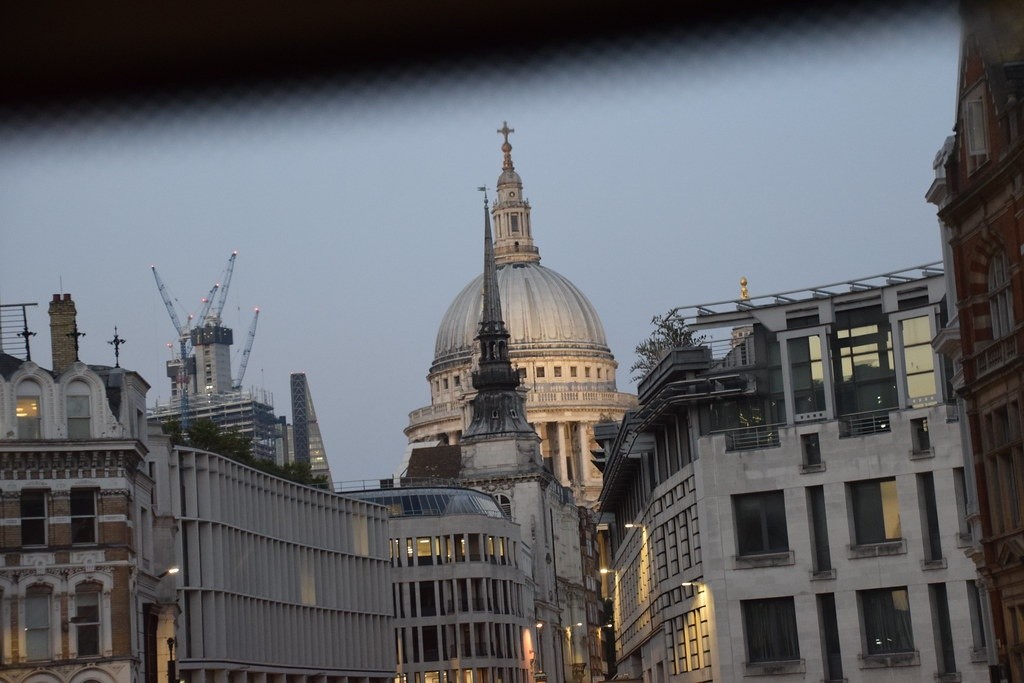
627 307 709 404
593 411 621 449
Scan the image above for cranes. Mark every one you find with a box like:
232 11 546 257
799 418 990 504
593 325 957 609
150 249 238 441
230 308 259 392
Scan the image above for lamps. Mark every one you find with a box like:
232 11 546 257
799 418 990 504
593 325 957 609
624 523 644 528
156 567 180 578
566 623 613 627
600 569 615 573
